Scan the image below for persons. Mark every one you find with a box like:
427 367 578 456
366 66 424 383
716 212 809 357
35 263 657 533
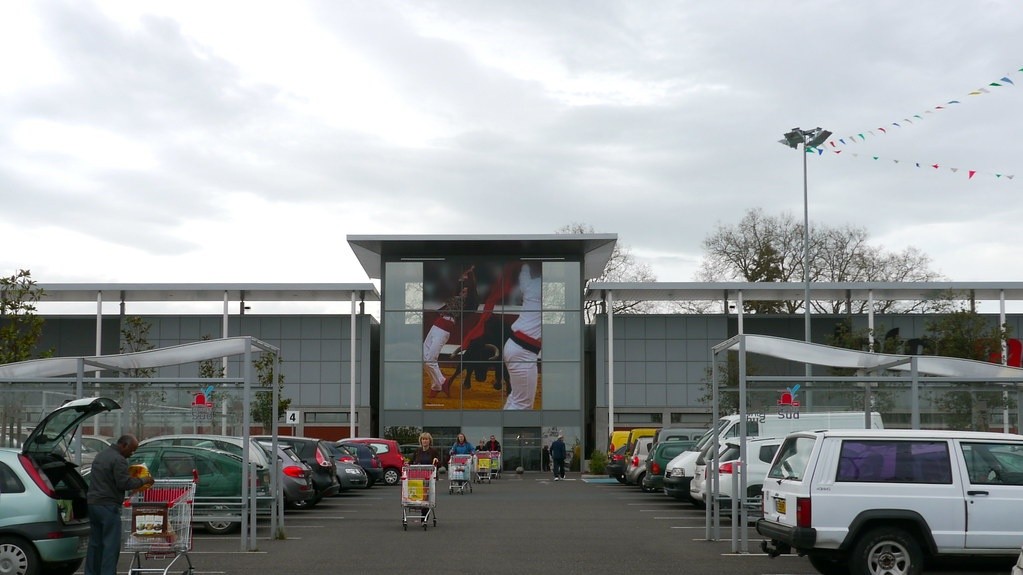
85 434 155 575
485 435 500 478
543 445 550 471
550 435 566 481
423 261 542 409
450 433 475 493
404 433 439 526
475 440 486 484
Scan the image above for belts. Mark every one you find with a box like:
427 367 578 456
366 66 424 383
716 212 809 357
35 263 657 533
509 333 539 354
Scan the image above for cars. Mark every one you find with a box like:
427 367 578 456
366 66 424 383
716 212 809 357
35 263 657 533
325 441 358 464
82 434 117 450
336 437 405 485
607 446 627 483
251 435 341 507
194 440 314 501
625 438 656 492
0 396 122 574
335 460 368 495
138 434 273 470
82 445 283 534
337 442 383 489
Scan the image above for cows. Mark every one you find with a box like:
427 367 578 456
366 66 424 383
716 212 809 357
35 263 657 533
447 315 512 397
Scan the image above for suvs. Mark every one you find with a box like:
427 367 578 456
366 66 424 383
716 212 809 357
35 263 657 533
400 444 422 461
702 437 785 524
755 428 1022 575
689 437 727 499
642 441 701 493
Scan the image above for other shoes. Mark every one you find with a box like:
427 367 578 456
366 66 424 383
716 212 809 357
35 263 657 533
554 478 558 480
427 388 439 398
457 488 461 492
482 479 485 482
490 476 494 478
442 378 449 395
561 475 565 480
421 515 428 526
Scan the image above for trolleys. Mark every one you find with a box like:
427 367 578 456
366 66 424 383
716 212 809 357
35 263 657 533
400 462 439 530
473 450 491 484
123 469 199 575
491 451 502 480
447 451 474 495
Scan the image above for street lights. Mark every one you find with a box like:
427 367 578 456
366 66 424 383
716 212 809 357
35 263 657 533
778 126 832 411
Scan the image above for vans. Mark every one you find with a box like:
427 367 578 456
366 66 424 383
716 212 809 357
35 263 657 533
607 431 631 459
625 429 658 465
653 428 708 443
663 410 885 500
0 427 99 476
